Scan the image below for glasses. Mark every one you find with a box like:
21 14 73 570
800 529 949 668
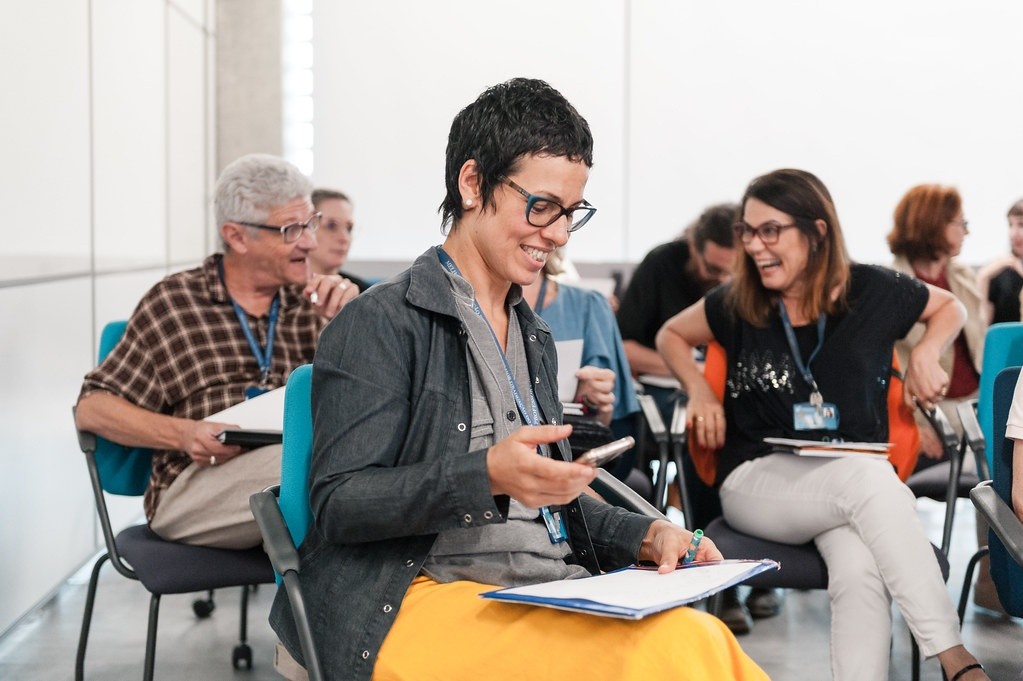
950 219 968 229
701 254 729 275
231 209 323 243
735 221 799 245
491 173 597 232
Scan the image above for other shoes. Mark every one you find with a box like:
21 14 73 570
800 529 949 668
746 586 786 615
719 586 754 631
975 583 1004 613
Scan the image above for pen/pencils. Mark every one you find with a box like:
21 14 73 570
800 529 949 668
682 529 702 564
311 290 317 304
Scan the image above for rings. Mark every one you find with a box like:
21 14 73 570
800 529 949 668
339 283 347 290
696 416 704 422
210 456 215 465
715 414 725 419
941 387 946 396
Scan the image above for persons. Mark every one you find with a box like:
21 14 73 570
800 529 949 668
1005 366 1023 523
268 79 770 681
887 182 1009 614
656 168 991 681
75 152 359 681
307 188 370 294
618 203 738 511
521 247 642 419
976 198 1023 326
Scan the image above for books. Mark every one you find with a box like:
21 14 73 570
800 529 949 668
215 431 283 447
762 438 890 459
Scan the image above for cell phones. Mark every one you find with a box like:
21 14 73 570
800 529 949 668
574 436 634 467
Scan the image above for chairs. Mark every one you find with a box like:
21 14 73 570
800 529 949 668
73 319 1023 681
